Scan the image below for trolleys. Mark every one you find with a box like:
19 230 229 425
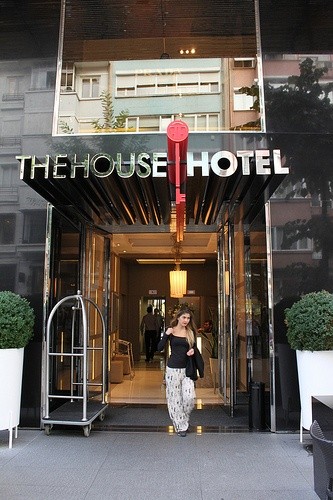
40 289 110 437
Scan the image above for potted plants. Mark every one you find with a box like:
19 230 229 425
284 289 333 443
0 290 35 448
199 304 218 390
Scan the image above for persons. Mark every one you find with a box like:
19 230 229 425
197 320 215 359
158 308 196 438
180 300 189 309
141 306 164 362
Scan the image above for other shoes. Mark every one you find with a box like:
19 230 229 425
145 359 149 361
151 357 153 359
178 430 188 440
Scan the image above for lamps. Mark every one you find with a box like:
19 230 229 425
169 264 187 298
160 0 173 59
